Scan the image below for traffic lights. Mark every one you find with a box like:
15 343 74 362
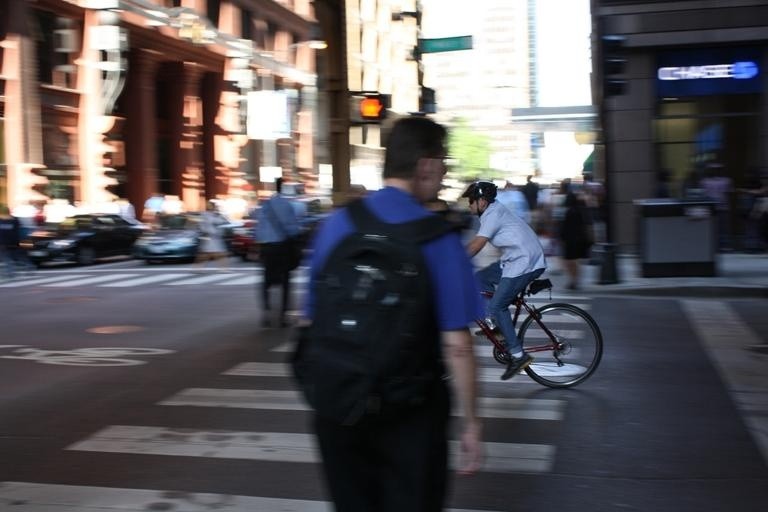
348 90 392 126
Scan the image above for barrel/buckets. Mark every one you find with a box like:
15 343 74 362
573 258 603 288
613 253 644 281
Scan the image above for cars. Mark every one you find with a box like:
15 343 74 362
16 211 153 269
221 204 325 264
0 213 46 251
131 210 209 265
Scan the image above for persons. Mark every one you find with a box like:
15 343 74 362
556 177 595 296
292 116 487 511
455 180 547 383
249 177 305 332
734 164 768 257
701 161 736 253
523 174 541 211
201 198 226 265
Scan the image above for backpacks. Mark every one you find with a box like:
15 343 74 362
291 198 462 423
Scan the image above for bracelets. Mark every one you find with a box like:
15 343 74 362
462 418 484 438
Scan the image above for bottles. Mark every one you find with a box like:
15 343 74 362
483 313 497 331
289 264 312 322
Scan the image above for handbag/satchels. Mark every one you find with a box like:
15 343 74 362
283 238 301 272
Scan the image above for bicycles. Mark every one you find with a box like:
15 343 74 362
475 278 603 388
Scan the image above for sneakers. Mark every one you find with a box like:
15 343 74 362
475 326 502 336
263 308 297 326
501 353 533 380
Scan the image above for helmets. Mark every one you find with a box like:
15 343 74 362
462 182 498 203
